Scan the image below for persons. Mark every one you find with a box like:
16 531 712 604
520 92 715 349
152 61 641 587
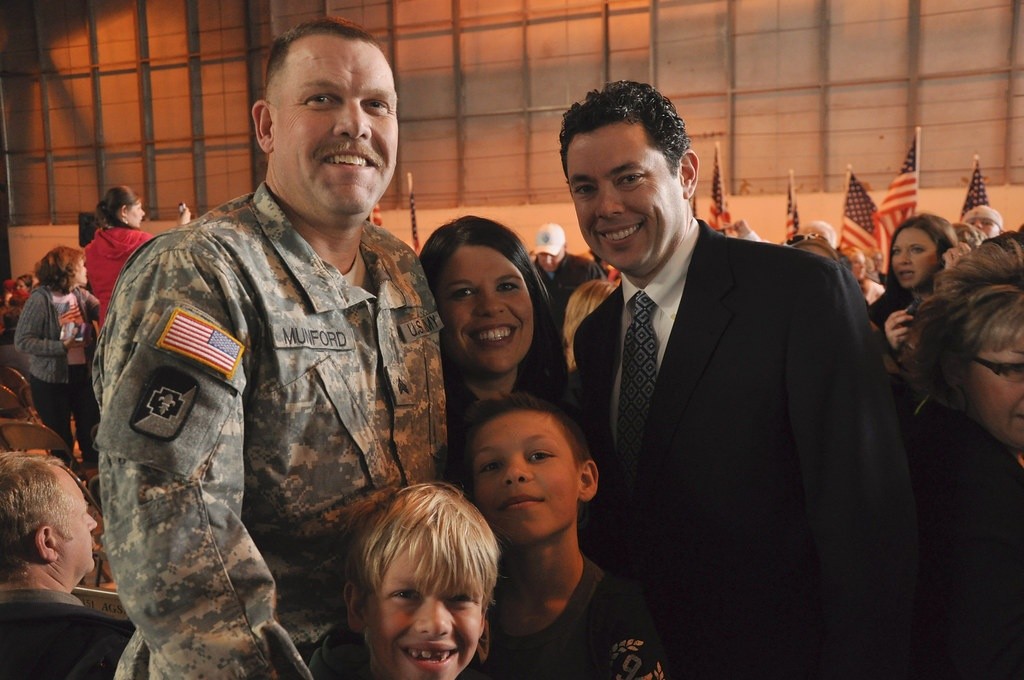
308 481 501 680
84 185 192 329
91 16 447 680
14 246 100 467
461 392 667 680
0 274 33 344
560 78 918 680
902 223 1024 680
725 205 1005 414
418 214 569 492
0 451 137 680
531 223 621 369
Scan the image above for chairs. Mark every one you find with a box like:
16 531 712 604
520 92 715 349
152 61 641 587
0 366 112 586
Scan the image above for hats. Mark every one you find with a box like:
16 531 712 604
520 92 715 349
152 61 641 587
535 224 565 256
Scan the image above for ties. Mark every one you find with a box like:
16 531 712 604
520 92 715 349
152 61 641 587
616 291 659 504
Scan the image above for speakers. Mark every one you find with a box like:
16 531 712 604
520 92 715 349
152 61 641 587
79 212 99 247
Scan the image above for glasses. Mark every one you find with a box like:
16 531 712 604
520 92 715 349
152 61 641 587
970 354 1024 382
967 217 1001 232
784 234 829 247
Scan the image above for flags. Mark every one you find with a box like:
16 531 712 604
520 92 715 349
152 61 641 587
960 159 989 221
873 135 918 271
709 147 731 231
837 173 879 248
786 182 799 238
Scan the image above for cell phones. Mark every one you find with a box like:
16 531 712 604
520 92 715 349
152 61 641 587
902 297 924 328
179 201 185 213
